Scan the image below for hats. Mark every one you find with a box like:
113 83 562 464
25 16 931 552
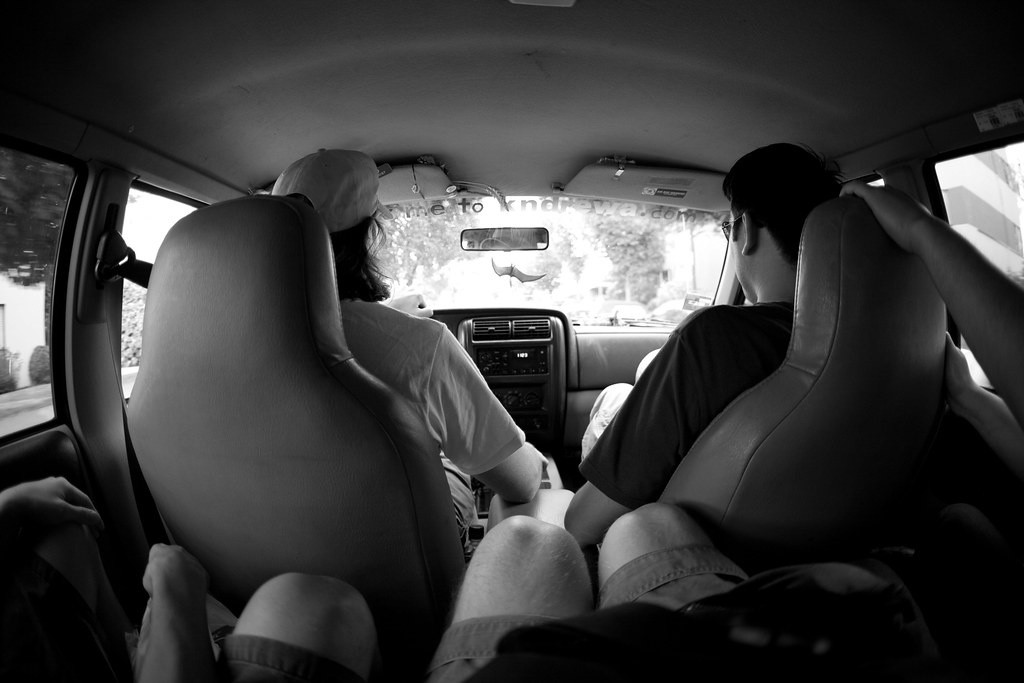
271 148 394 233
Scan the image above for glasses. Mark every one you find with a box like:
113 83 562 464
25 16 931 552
722 215 764 241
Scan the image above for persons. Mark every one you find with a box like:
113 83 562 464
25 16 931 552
427 503 950 683
0 476 382 683
563 139 842 548
834 177 1024 683
272 149 549 547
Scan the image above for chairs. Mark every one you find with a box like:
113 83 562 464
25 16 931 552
129 191 470 682
665 203 946 575
